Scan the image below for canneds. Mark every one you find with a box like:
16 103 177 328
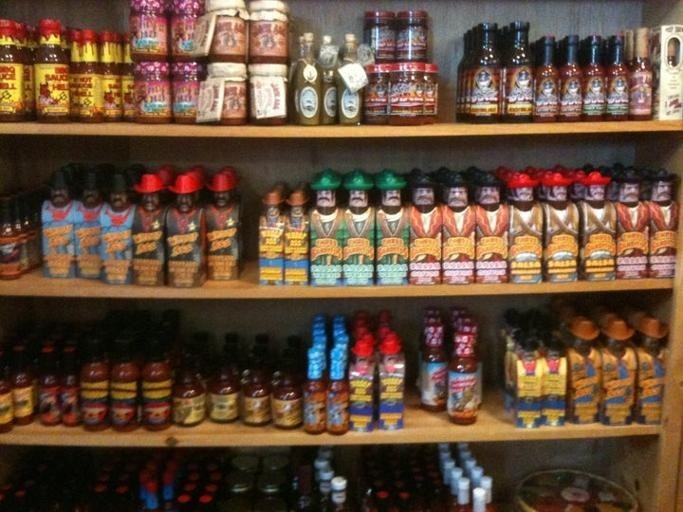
206 61 247 126
249 0 290 64
363 9 441 125
247 61 289 126
128 0 170 62
79 352 111 430
172 61 204 125
133 62 173 124
139 352 172 432
173 331 304 430
61 344 81 428
110 349 140 431
204 0 249 64
170 0 206 62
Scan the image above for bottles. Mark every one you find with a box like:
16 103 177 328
78 169 105 279
341 34 365 127
311 171 344 286
410 173 441 284
454 22 507 124
67 28 79 124
449 306 480 424
166 176 206 289
99 30 120 123
20 22 38 122
258 190 284 286
305 308 331 437
618 174 650 280
121 30 141 122
442 170 476 284
0 19 26 122
420 306 447 412
509 170 544 283
206 175 246 281
0 197 39 281
0 441 504 512
646 175 677 280
584 35 610 122
536 35 560 122
0 349 12 433
294 29 323 127
346 170 375 286
103 172 134 285
477 169 509 285
604 319 634 425
506 18 533 124
568 321 605 426
376 169 410 284
328 310 355 436
634 315 669 429
542 338 569 426
609 34 631 122
134 175 169 288
625 28 656 122
351 306 377 435
37 345 67 428
561 32 585 122
498 306 544 429
284 192 311 285
320 34 340 126
10 347 36 426
544 173 579 282
579 172 618 285
32 17 70 123
79 28 100 123
375 308 408 431
40 170 77 277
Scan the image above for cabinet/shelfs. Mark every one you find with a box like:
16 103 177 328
1 118 682 510
